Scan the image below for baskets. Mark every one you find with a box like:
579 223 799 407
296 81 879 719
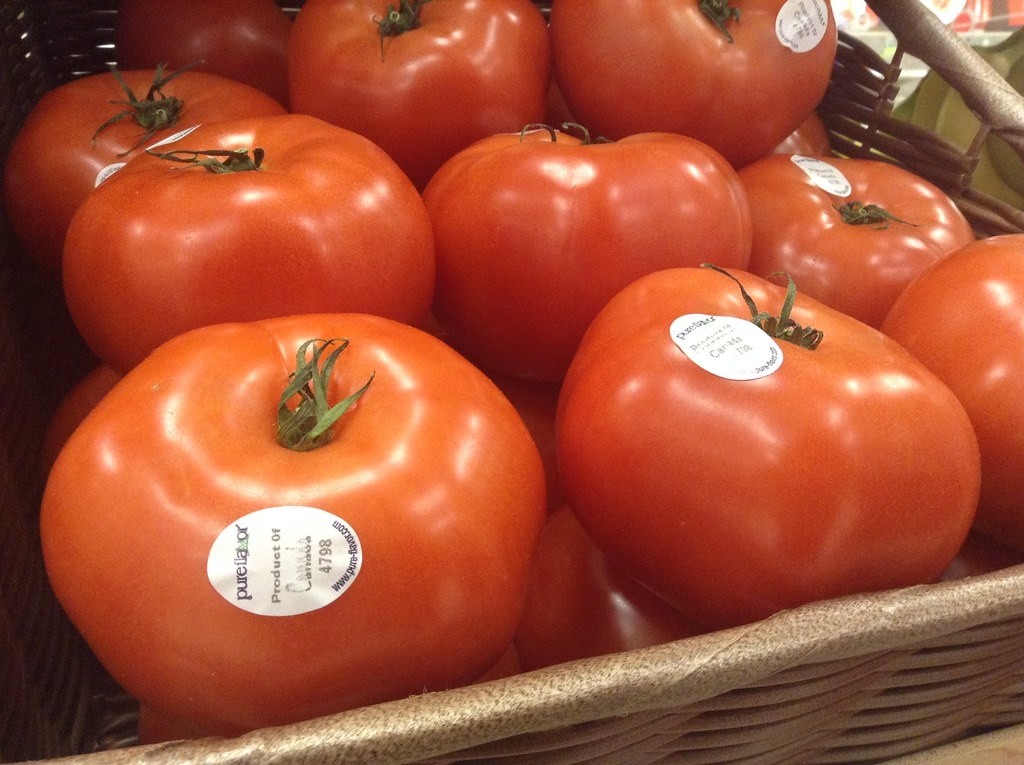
0 1 1024 765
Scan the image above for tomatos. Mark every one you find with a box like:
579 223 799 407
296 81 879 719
7 1 1024 743
